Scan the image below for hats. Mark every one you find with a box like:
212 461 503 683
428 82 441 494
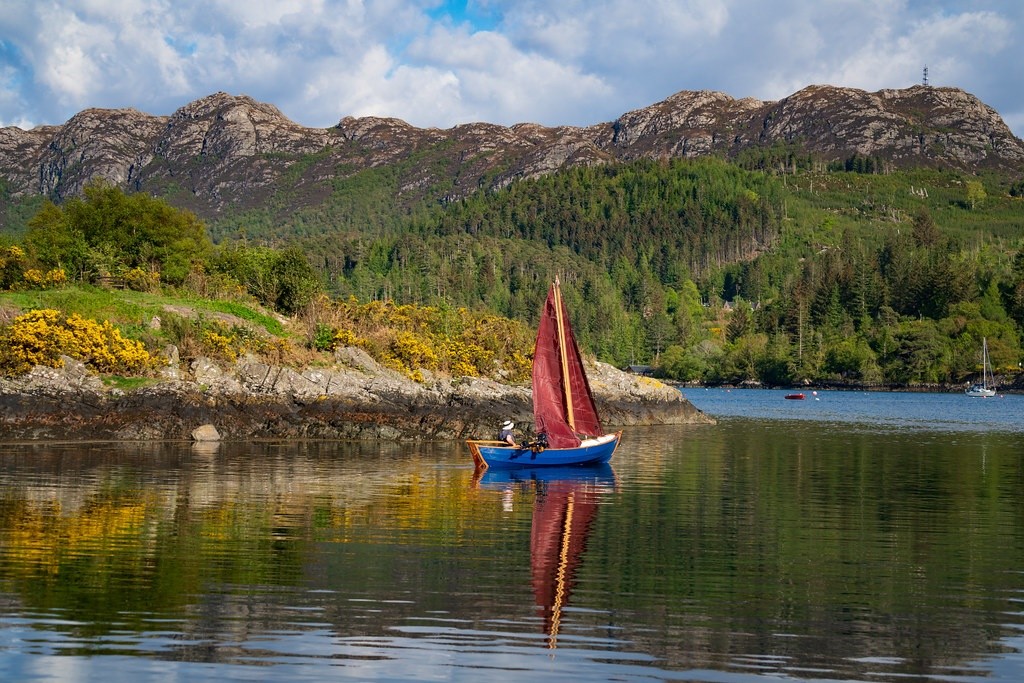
501 420 514 431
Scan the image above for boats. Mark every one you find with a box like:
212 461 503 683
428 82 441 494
784 393 806 399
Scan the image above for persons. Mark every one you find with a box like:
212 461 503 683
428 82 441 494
521 434 547 449
498 421 521 448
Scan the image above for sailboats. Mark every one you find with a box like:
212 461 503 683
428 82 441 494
465 276 624 470
965 337 997 397
470 462 623 658
